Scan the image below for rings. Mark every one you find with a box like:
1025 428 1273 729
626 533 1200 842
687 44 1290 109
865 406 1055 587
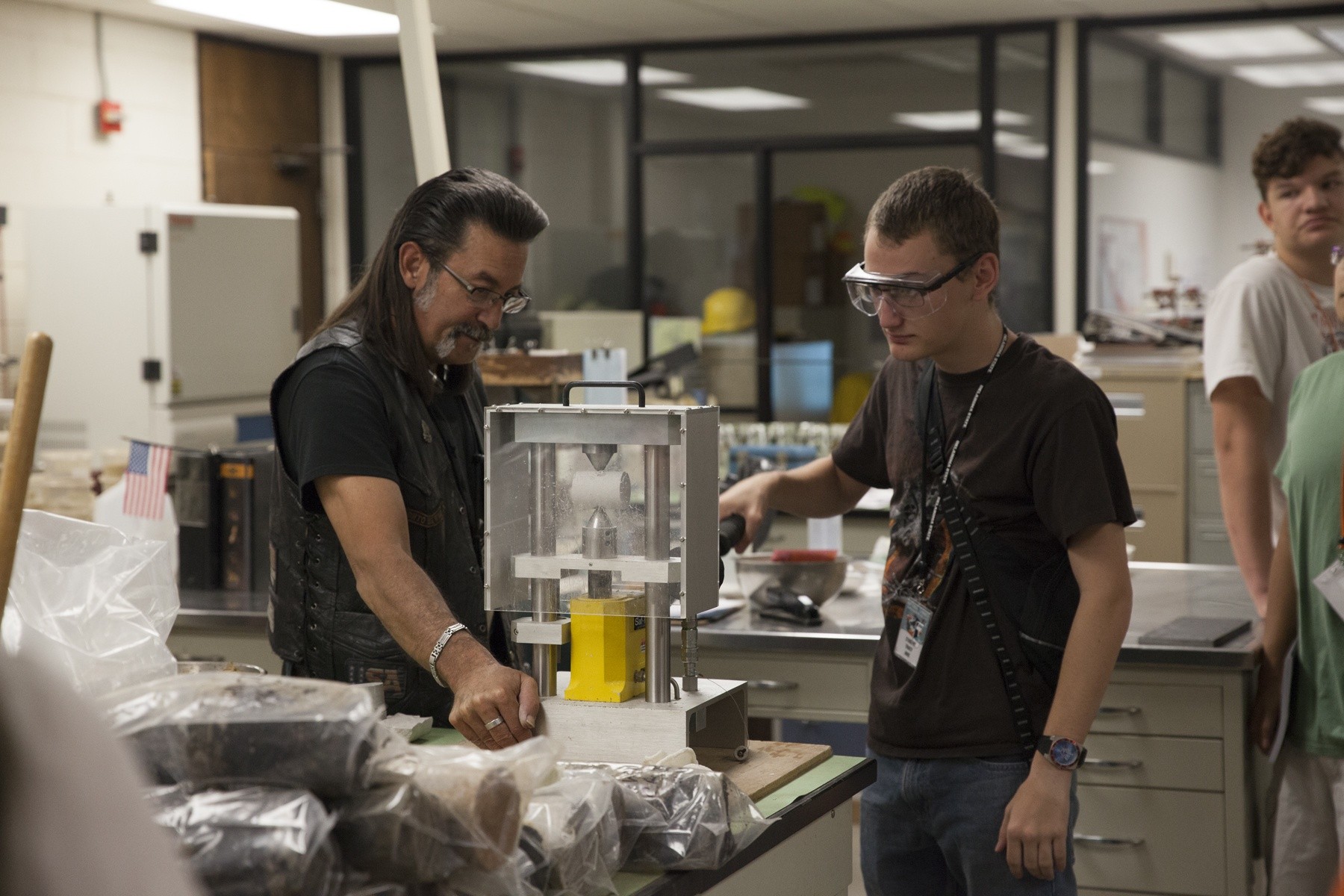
485 716 503 731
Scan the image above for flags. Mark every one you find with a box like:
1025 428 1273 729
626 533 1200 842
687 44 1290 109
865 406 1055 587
122 441 170 521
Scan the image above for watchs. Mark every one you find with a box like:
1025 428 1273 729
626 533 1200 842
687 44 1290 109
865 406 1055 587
1035 734 1087 771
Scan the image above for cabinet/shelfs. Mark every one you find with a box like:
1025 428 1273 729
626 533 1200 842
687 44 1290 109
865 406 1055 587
1032 334 1237 566
670 562 1263 896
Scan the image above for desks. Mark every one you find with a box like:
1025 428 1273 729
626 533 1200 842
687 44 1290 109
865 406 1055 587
408 728 877 896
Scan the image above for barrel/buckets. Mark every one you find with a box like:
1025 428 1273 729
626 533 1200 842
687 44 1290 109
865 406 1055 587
771 341 835 419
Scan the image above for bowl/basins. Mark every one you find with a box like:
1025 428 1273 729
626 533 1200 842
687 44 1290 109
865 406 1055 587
736 554 851 608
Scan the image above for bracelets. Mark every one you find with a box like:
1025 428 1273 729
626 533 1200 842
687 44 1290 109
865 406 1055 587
430 623 471 690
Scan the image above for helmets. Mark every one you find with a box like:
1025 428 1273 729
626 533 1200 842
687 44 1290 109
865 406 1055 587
700 288 757 335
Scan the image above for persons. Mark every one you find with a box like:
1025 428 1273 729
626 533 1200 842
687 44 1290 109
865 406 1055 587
1201 116 1344 896
715 166 1135 896
268 170 551 751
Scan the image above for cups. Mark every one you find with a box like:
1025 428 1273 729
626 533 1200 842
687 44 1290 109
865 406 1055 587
808 516 844 556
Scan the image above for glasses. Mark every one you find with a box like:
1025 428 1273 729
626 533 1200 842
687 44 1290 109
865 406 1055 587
841 253 988 322
418 247 532 314
1331 246 1344 266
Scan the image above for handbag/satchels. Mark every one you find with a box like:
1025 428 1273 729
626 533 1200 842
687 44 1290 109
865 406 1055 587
0 503 211 895
